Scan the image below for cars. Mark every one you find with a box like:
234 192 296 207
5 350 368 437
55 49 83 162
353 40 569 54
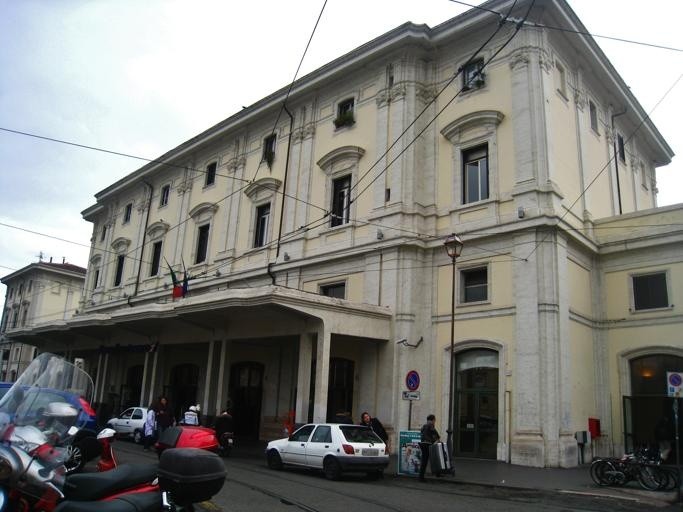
459 414 498 442
260 423 391 482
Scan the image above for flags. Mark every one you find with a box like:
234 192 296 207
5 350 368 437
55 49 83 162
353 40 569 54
182 270 189 297
166 261 181 302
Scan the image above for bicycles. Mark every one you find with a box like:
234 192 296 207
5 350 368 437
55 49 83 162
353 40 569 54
588 443 679 493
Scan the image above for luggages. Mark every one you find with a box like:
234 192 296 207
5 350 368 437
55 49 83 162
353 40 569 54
429 440 451 475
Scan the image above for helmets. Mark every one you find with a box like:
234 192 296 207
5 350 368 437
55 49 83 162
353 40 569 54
189 406 197 412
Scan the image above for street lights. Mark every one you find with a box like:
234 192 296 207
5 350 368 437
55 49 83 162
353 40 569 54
444 231 465 457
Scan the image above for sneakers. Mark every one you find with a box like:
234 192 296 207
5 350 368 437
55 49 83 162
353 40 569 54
143 448 152 452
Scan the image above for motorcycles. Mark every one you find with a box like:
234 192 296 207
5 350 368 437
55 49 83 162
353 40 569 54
0 349 236 511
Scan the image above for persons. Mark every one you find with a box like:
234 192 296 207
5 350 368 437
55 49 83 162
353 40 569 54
360 412 389 445
154 398 176 450
143 401 158 450
417 414 445 483
653 412 673 463
214 409 235 450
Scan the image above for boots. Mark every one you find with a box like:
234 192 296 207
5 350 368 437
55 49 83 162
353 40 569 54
419 473 428 482
